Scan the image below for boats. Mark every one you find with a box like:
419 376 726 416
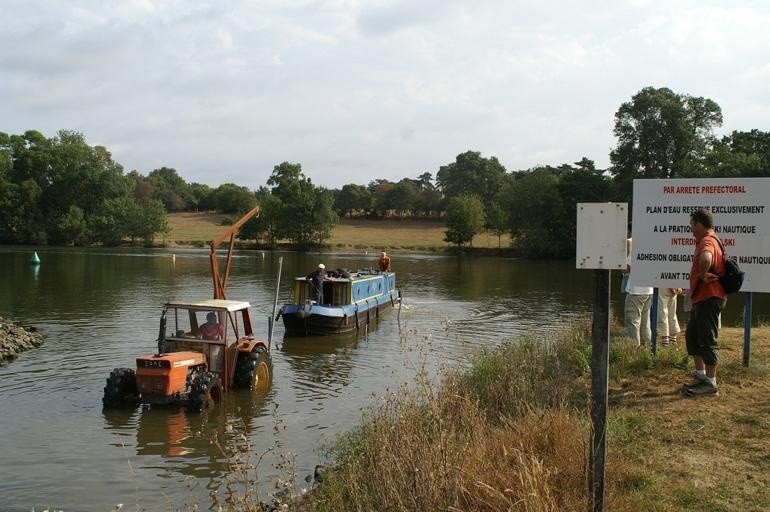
282 269 399 338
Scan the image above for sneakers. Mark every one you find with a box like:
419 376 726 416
684 380 720 397
683 374 705 389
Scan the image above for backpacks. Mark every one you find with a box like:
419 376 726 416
708 235 746 294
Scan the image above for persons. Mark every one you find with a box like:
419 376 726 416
379 252 391 272
681 208 728 397
306 264 330 304
197 313 224 340
657 288 686 347
620 236 654 351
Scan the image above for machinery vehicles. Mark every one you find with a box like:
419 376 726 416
104 206 274 412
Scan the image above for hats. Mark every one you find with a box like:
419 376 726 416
380 252 386 259
317 264 325 269
